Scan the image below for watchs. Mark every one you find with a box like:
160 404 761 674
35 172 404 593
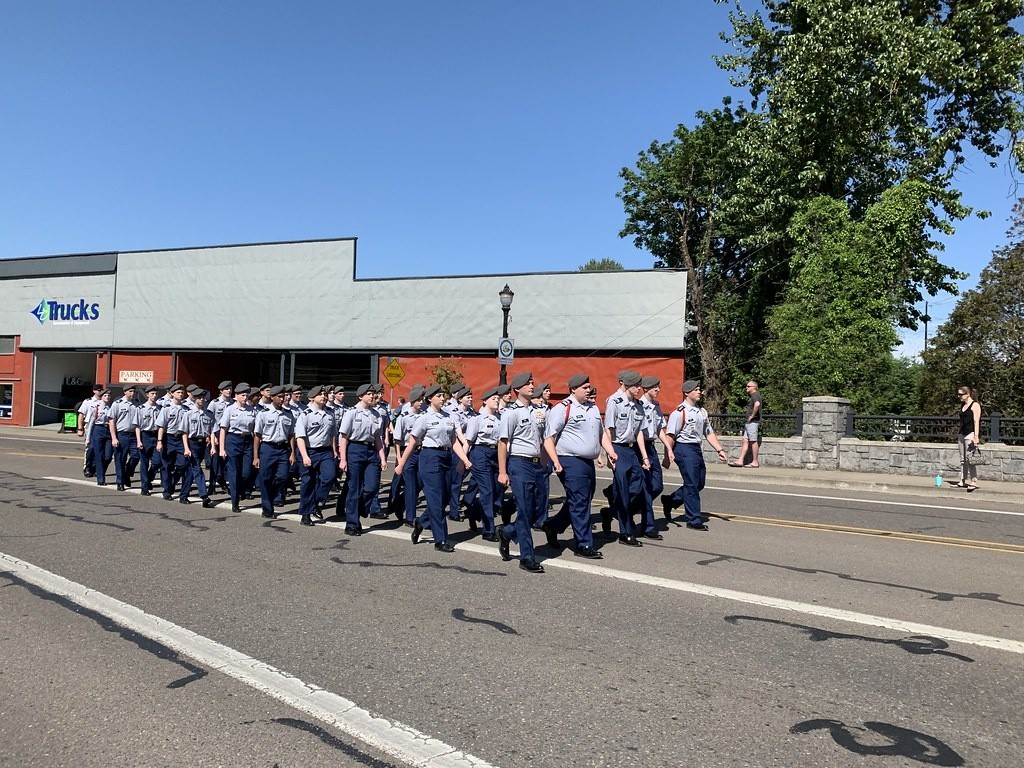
77 428 84 431
717 448 725 454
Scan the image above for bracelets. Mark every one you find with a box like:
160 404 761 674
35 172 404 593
642 456 648 461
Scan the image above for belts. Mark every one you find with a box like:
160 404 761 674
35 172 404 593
190 438 206 442
511 455 541 463
400 445 421 450
94 423 109 427
476 444 497 449
349 440 372 445
423 446 451 450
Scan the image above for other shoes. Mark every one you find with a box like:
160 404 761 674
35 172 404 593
344 527 361 536
262 489 326 526
164 484 255 513
729 461 743 467
448 498 512 542
661 495 673 521
371 497 423 544
591 488 614 536
744 463 759 468
85 470 153 495
687 522 708 530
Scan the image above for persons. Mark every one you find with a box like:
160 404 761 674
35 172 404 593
542 374 618 561
83 389 114 486
950 386 982 494
107 384 140 491
132 371 552 574
604 369 675 548
728 380 763 469
77 383 104 473
584 387 605 532
661 380 729 532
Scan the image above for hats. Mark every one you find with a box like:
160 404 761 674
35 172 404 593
218 381 345 398
408 382 471 402
617 370 701 393
479 373 551 399
356 383 384 397
568 374 596 395
92 382 207 398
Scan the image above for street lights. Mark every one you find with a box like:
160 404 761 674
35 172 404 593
498 282 514 386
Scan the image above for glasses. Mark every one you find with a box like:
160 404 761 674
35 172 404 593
958 392 967 396
747 386 756 388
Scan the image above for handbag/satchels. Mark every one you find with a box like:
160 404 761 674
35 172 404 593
967 449 988 465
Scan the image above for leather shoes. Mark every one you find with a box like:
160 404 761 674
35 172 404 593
619 534 643 547
541 519 561 548
434 541 455 551
520 557 544 573
574 545 602 559
641 528 664 540
495 525 511 561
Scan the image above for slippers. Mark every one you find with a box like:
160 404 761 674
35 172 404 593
967 484 980 491
954 482 968 487
